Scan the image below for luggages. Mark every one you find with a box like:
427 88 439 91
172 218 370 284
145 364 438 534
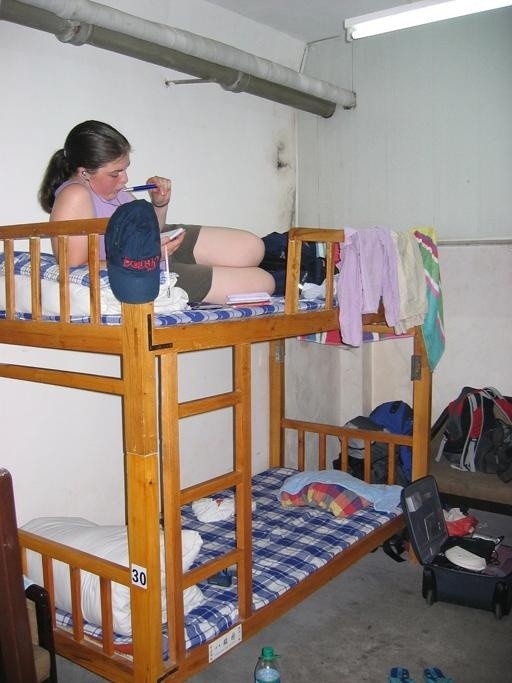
399 474 512 620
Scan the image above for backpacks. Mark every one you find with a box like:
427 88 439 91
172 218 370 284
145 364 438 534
262 229 317 296
332 399 412 562
430 386 512 484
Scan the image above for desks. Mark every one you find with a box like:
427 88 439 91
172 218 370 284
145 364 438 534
427 463 512 518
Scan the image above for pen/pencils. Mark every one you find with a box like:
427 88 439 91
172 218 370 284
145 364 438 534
122 184 157 192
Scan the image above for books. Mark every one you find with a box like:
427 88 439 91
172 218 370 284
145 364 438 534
224 292 272 308
160 226 186 241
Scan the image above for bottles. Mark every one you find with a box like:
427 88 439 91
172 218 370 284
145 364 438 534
254 646 282 683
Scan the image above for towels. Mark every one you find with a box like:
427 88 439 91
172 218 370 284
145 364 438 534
281 468 404 515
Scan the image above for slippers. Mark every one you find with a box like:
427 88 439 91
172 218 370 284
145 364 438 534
388 667 415 683
422 666 453 683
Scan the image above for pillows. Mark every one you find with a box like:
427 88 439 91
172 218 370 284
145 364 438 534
279 482 371 518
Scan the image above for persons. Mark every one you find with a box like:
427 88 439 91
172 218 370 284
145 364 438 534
39 119 277 306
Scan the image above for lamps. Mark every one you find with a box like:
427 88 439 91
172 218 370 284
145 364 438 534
343 0 512 44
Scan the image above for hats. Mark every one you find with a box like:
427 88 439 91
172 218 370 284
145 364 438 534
104 199 161 304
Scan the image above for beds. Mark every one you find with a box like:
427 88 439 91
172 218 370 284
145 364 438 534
0 464 430 683
1 221 439 464
